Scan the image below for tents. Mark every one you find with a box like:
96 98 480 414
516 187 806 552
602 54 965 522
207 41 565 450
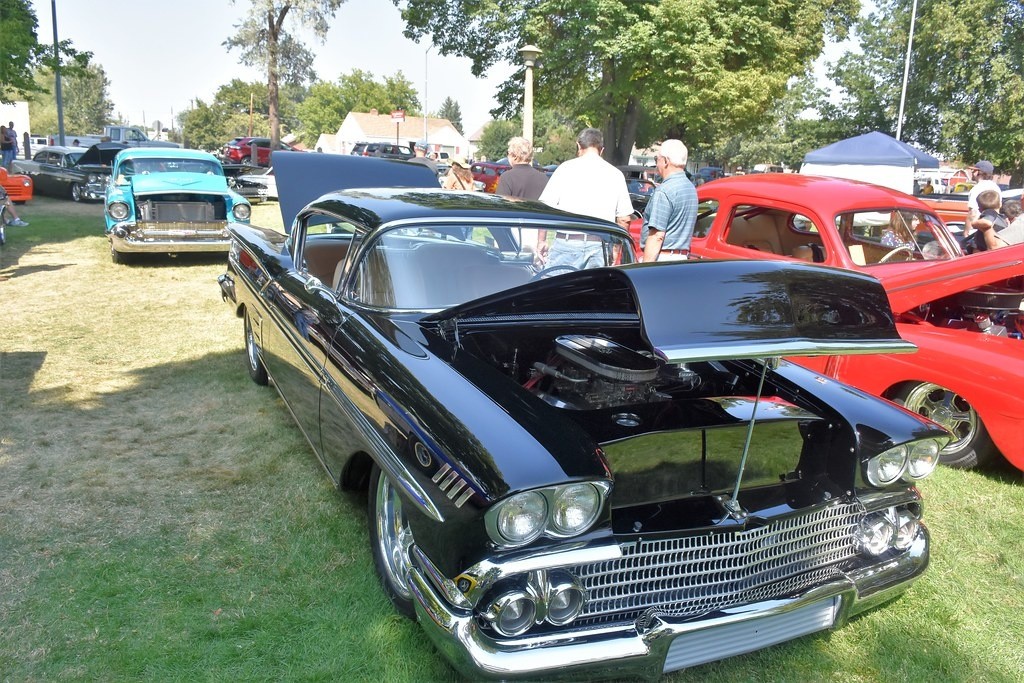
798 131 943 225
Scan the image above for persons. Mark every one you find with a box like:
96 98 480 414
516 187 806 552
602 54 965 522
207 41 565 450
0 185 30 227
969 190 1024 252
639 139 699 261
496 136 548 255
913 179 922 196
880 209 919 252
407 140 439 179
442 161 477 193
964 161 1001 237
73 139 80 147
533 128 635 278
0 121 20 169
924 180 934 194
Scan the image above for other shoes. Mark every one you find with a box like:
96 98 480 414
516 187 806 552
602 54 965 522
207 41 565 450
6 219 29 227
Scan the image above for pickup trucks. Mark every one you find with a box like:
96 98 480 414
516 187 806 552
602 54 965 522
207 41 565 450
46 125 181 148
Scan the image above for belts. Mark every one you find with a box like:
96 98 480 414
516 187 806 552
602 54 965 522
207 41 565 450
556 232 600 242
659 249 689 254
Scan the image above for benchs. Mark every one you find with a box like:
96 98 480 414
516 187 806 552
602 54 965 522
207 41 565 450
302 240 532 304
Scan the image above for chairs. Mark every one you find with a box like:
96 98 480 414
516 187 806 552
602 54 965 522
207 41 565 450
335 251 427 309
793 245 826 261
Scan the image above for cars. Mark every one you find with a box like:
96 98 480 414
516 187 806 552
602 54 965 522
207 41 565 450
609 171 1024 473
793 187 1024 256
348 141 784 221
216 149 956 683
0 165 34 204
163 136 312 205
17 137 47 156
8 142 132 202
104 147 253 264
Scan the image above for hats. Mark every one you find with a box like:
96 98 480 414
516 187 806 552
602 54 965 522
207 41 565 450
448 154 472 169
415 140 429 151
969 161 994 174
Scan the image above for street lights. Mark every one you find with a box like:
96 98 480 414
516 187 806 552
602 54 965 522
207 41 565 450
516 43 545 167
